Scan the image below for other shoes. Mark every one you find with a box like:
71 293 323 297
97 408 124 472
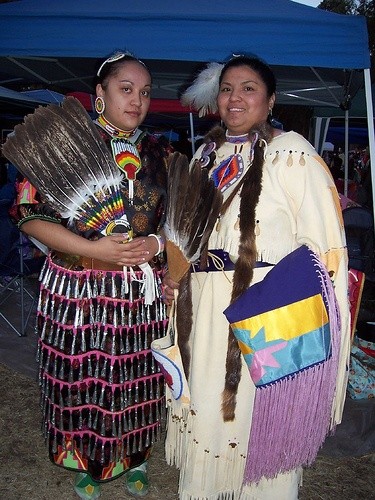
126 461 149 496
74 472 101 500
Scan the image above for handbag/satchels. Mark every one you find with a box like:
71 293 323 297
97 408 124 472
150 288 191 407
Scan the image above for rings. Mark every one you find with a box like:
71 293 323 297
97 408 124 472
162 285 169 290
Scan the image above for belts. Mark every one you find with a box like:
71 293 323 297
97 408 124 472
56 249 161 272
190 249 274 273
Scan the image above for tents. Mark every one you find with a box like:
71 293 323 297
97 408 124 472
0 0 375 216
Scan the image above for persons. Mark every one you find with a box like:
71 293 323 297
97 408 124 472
325 140 371 180
10 49 187 500
162 54 352 499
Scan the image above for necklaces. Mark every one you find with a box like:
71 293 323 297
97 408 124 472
224 126 249 143
98 113 137 138
